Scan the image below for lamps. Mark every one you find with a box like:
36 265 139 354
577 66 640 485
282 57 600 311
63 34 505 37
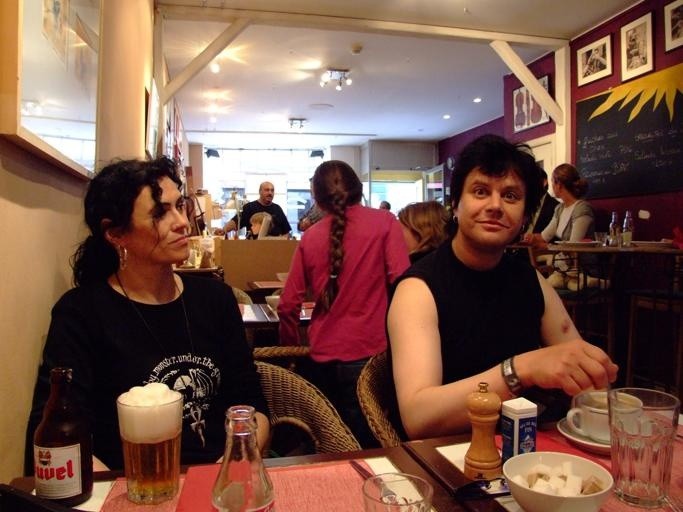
320 68 352 92
288 117 308 130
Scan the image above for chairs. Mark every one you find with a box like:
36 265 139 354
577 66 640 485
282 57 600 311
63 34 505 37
355 349 409 447
255 346 311 357
252 359 362 460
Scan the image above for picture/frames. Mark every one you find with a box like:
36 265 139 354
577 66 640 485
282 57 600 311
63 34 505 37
42 1 70 62
663 0 683 53
576 32 613 88
512 73 550 134
620 10 655 83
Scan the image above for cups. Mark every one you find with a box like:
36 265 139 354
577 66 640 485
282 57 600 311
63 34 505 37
362 471 432 512
593 230 607 247
606 386 680 509
116 389 183 503
565 391 642 444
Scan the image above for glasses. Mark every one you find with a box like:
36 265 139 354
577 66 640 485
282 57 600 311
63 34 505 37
454 478 511 500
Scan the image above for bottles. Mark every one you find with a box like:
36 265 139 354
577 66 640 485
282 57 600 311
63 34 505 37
30 366 94 508
621 211 633 248
608 211 621 246
224 230 238 240
211 405 277 512
460 380 503 485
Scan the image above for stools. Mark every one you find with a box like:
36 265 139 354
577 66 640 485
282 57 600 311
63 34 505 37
546 291 683 414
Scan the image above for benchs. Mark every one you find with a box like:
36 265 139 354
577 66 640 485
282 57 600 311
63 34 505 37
582 192 683 241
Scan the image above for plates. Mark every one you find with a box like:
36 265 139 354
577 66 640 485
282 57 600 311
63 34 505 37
629 240 667 249
551 240 596 248
553 418 610 451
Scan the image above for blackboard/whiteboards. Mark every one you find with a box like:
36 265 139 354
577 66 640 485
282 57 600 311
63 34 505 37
574 62 683 200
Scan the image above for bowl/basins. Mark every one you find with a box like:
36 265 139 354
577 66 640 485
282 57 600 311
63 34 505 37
265 295 280 311
500 452 615 511
275 272 290 282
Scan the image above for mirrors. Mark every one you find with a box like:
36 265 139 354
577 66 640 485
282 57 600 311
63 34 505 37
0 1 105 182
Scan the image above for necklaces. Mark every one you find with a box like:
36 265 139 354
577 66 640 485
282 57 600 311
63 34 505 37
115 273 206 448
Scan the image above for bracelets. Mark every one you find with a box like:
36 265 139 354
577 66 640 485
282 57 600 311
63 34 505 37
502 357 524 396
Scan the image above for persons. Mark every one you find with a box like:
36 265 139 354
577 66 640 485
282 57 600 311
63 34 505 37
525 163 611 291
398 200 450 265
385 134 619 443
214 182 291 236
249 211 274 240
520 167 560 244
23 158 271 477
297 178 328 231
380 202 391 210
277 160 412 454
